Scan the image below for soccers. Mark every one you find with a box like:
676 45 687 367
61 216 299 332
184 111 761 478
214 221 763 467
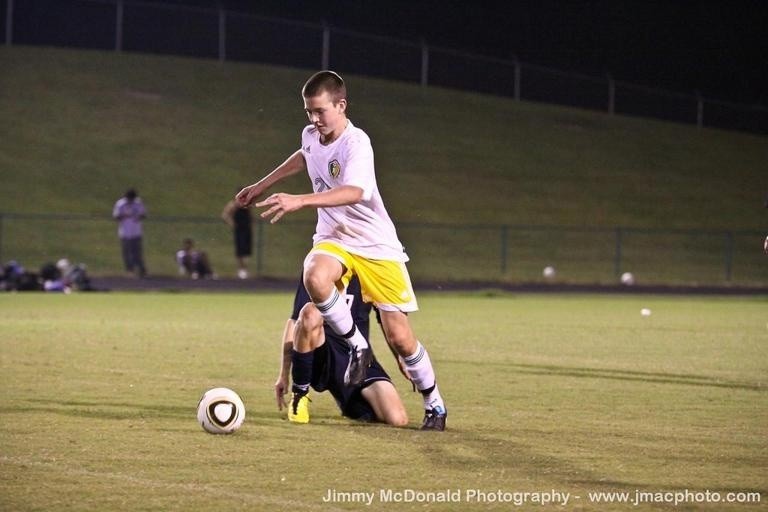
197 388 246 435
543 267 556 279
620 272 633 285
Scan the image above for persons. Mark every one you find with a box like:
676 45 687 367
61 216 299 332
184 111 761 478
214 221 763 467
274 268 415 426
176 238 218 280
235 71 447 431
0 258 111 294
113 189 151 280
222 187 253 279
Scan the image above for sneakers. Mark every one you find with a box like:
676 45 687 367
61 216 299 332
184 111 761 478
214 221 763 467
343 343 373 386
288 385 312 423
421 407 447 431
